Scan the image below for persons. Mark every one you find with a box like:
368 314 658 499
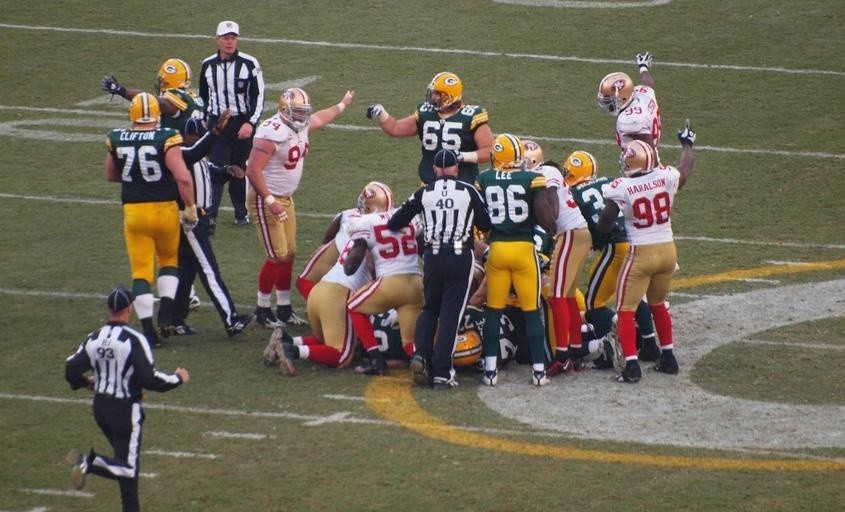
65 287 190 512
264 120 698 391
199 21 265 234
244 87 354 328
366 72 496 180
161 107 257 338
599 48 663 171
100 58 206 311
104 92 199 348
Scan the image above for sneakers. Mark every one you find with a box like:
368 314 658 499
208 217 215 233
233 216 249 224
67 449 88 489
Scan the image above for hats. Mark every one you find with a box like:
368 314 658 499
216 20 240 37
183 119 207 136
106 287 136 311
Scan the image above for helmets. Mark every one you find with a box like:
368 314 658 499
156 59 191 91
130 92 160 123
279 88 312 132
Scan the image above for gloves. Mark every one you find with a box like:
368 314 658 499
677 118 695 145
182 205 198 231
636 51 653 71
103 75 125 95
